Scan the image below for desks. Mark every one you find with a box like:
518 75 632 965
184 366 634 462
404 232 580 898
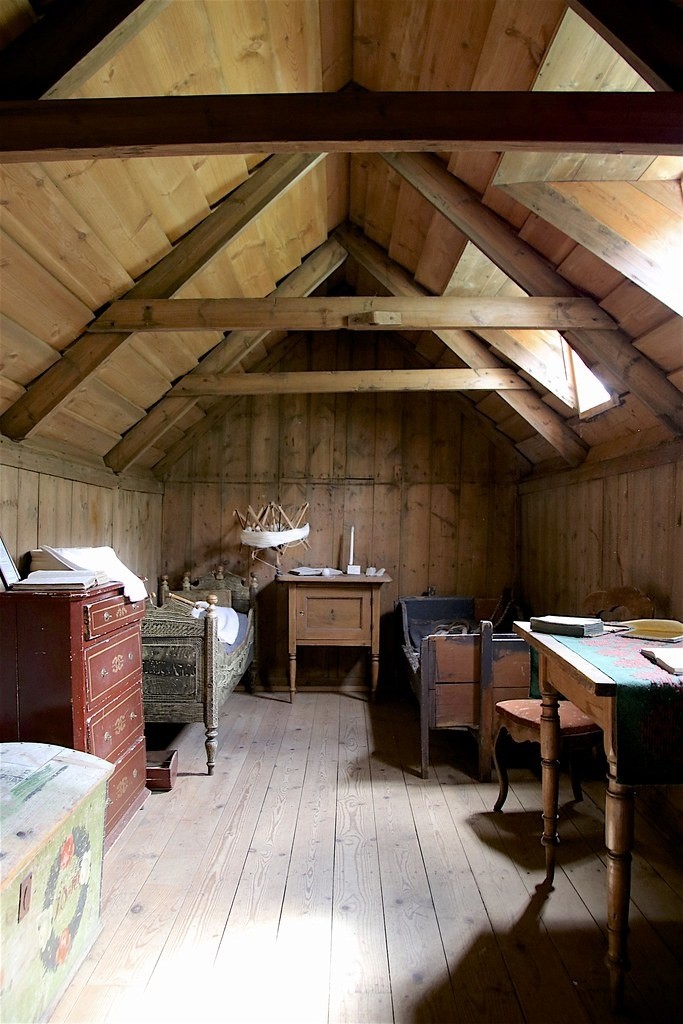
513 620 683 1017
275 572 392 704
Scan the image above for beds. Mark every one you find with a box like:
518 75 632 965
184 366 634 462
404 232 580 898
396 594 546 779
139 564 259 775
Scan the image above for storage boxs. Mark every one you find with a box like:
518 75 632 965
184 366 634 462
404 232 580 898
145 750 179 791
0 743 118 1024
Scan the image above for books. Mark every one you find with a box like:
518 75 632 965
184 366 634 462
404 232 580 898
530 616 629 637
611 619 683 643
640 646 683 676
11 570 112 590
289 566 344 576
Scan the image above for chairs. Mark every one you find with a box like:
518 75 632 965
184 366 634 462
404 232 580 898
491 585 656 816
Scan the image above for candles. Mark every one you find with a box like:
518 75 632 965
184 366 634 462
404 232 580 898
349 526 355 566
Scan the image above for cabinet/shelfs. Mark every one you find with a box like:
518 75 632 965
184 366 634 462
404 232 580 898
0 578 151 857
